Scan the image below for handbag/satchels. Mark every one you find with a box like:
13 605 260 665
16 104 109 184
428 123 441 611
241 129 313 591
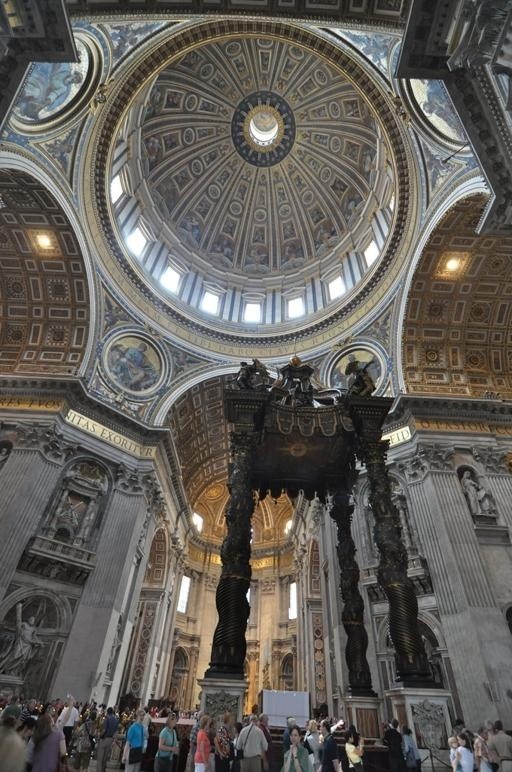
128 746 144 764
89 733 97 749
236 748 246 759
353 761 366 772
404 746 419 768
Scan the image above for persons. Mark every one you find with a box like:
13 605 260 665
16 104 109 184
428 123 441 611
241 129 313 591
111 342 148 389
460 471 484 516
346 355 380 388
1 693 512 772
1 616 44 677
420 85 461 128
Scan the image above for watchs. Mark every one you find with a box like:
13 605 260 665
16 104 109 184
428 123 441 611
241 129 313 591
20 58 83 105
